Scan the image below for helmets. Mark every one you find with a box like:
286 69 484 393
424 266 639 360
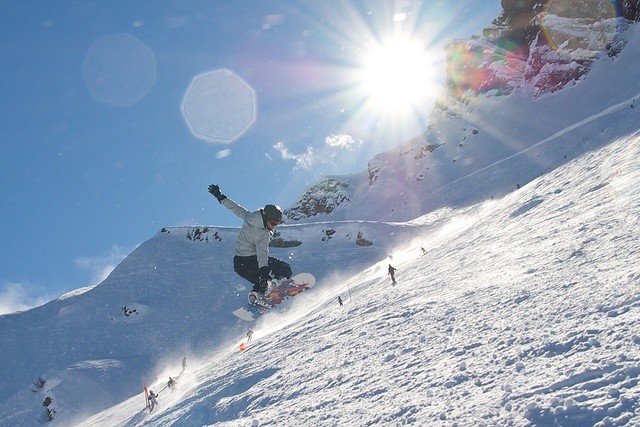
263 204 282 228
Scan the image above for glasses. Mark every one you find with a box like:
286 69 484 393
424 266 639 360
269 218 280 226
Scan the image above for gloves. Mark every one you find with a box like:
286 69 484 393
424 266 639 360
259 266 272 288
208 184 227 204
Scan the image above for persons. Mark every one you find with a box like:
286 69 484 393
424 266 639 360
387 264 397 284
180 357 187 375
247 328 254 342
339 296 343 306
167 377 177 393
208 183 292 294
421 247 427 254
148 390 158 413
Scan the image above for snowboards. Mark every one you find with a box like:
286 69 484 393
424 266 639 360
232 272 316 321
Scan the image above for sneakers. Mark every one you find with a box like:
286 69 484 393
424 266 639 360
271 279 282 288
247 291 259 306
